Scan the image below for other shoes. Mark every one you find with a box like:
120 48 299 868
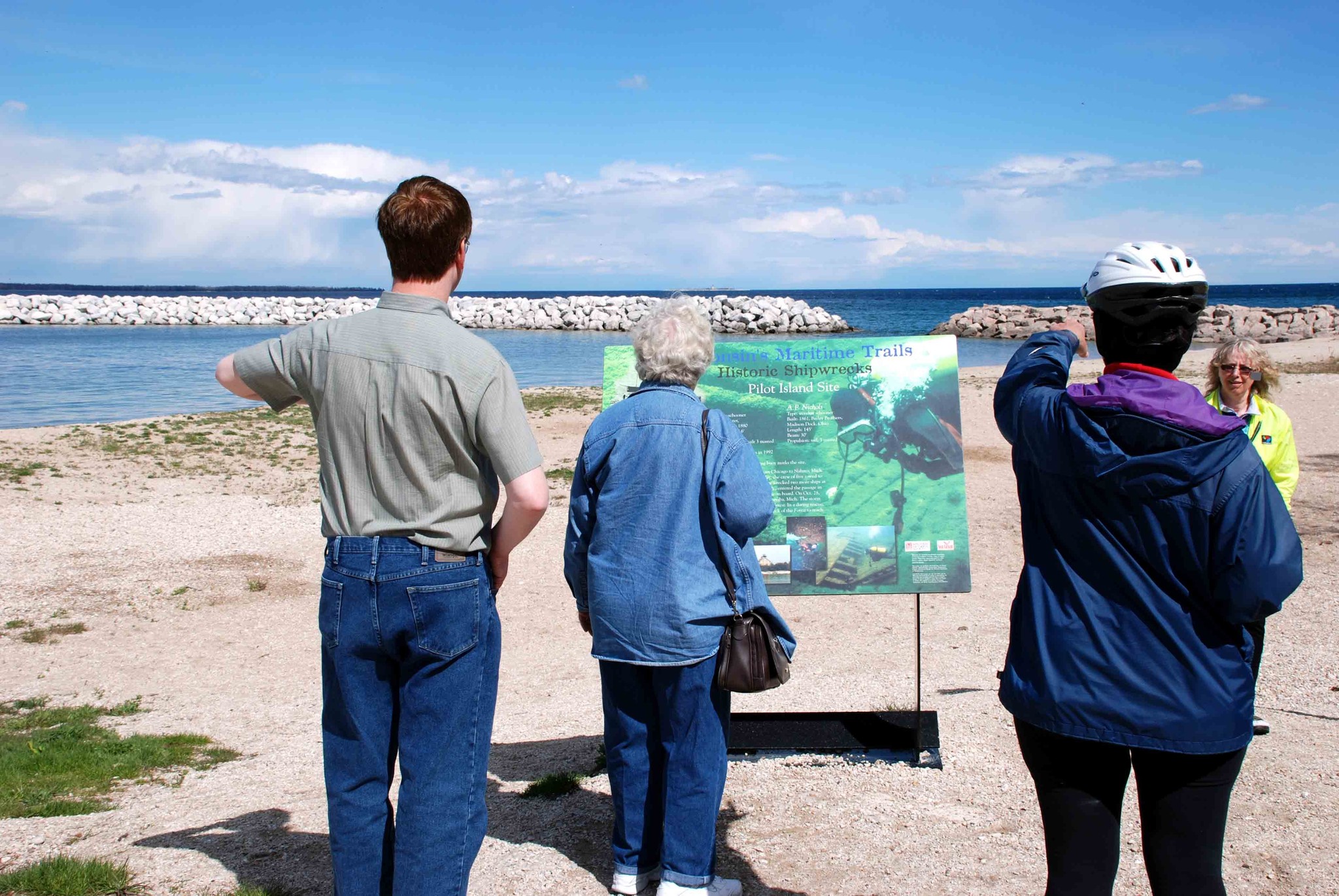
1252 715 1271 734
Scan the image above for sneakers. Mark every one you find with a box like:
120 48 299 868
657 876 740 896
612 867 660 895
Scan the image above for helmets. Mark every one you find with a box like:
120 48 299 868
1079 240 1210 314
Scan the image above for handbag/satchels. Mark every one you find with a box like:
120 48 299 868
717 612 790 693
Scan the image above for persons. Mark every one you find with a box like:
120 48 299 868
991 244 1305 896
561 309 786 896
1203 339 1299 735
214 178 551 896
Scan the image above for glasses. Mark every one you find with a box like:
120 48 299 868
1220 363 1252 375
